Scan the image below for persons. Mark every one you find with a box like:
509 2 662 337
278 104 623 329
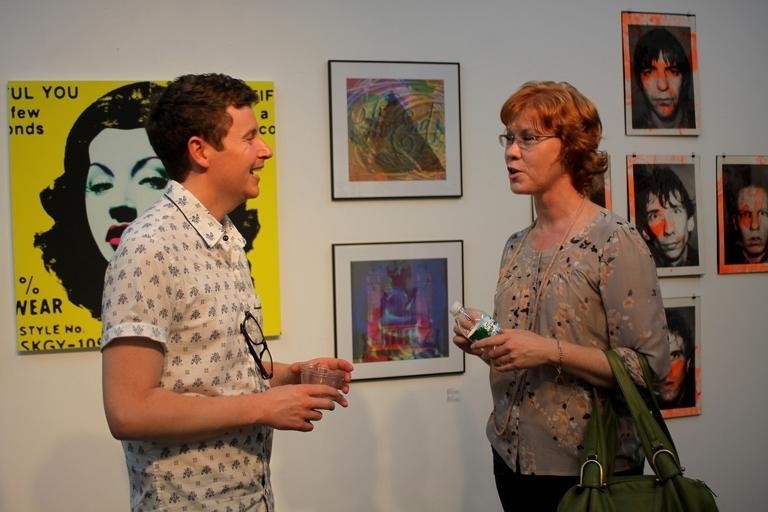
724 165 768 264
452 78 673 512
634 165 698 266
32 81 262 322
656 307 695 409
633 28 696 128
99 72 355 511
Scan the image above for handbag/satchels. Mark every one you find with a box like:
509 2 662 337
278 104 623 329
557 475 719 512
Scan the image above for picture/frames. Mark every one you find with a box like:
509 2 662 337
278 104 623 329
331 239 468 382
622 9 700 137
329 61 463 201
714 153 768 274
626 154 705 278
648 296 702 419
532 153 612 224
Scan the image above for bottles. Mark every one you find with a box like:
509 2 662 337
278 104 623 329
448 300 529 379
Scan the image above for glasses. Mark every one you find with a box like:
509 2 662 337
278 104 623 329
240 311 273 380
499 135 560 147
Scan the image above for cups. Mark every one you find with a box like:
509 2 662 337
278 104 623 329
299 360 344 390
